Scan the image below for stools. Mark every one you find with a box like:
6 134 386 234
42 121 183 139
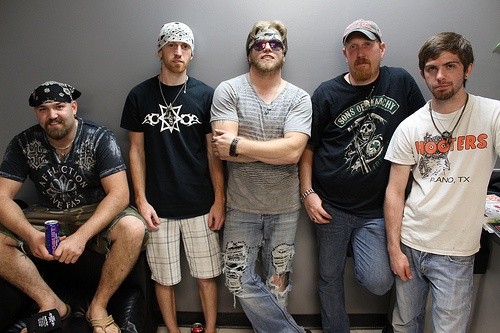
0 245 158 333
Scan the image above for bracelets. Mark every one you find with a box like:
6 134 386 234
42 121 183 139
229 137 240 158
301 188 315 202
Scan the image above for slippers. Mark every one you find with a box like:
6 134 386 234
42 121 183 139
85 311 121 333
21 304 71 333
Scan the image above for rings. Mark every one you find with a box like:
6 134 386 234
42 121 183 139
313 218 316 220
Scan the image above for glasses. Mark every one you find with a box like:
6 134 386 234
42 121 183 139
248 39 284 56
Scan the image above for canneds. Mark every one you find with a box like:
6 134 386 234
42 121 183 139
192 322 203 332
44 220 60 255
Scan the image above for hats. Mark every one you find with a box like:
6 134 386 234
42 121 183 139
343 19 382 47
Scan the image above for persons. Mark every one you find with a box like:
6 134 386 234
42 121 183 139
210 25 313 333
0 80 150 333
298 19 427 333
382 32 500 333
120 22 226 333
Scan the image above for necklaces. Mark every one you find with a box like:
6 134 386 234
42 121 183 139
429 93 469 144
159 76 187 115
348 74 376 100
50 139 73 163
